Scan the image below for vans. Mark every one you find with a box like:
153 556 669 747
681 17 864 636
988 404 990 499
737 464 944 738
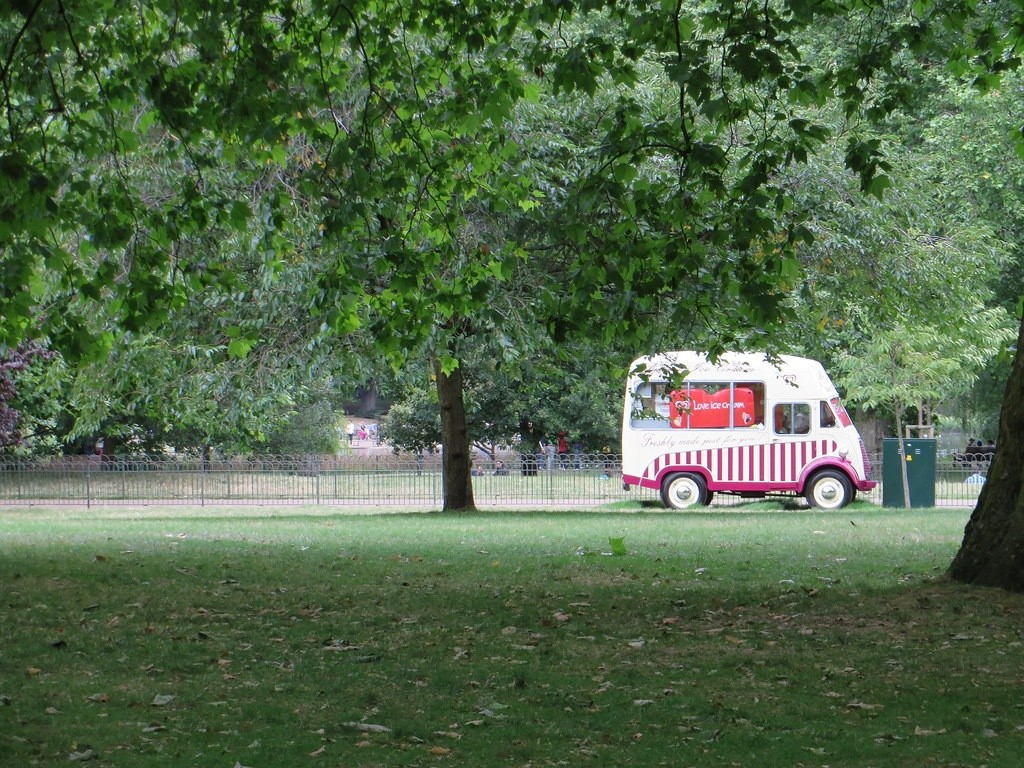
621 349 877 509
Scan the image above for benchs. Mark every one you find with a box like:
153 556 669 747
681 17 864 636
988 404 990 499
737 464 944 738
338 439 368 454
953 446 997 467
373 440 420 455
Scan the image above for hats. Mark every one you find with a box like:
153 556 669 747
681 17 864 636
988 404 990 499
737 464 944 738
969 438 975 441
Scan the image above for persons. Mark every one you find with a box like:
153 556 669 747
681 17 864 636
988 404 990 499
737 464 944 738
345 419 381 445
967 438 995 449
472 465 485 476
537 430 584 471
492 460 509 477
